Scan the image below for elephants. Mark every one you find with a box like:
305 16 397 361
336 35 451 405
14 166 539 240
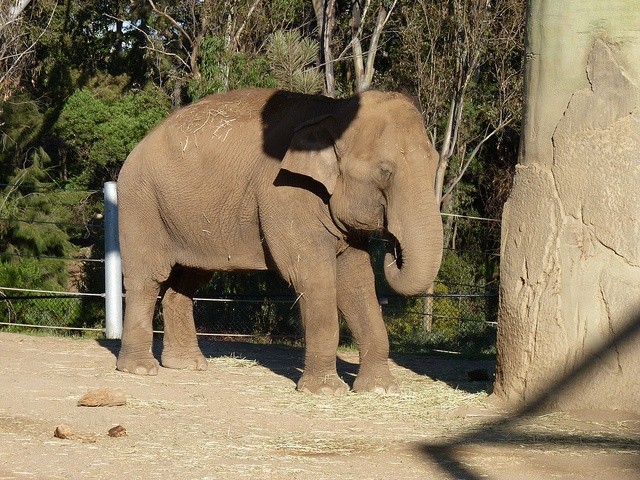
115 86 445 397
